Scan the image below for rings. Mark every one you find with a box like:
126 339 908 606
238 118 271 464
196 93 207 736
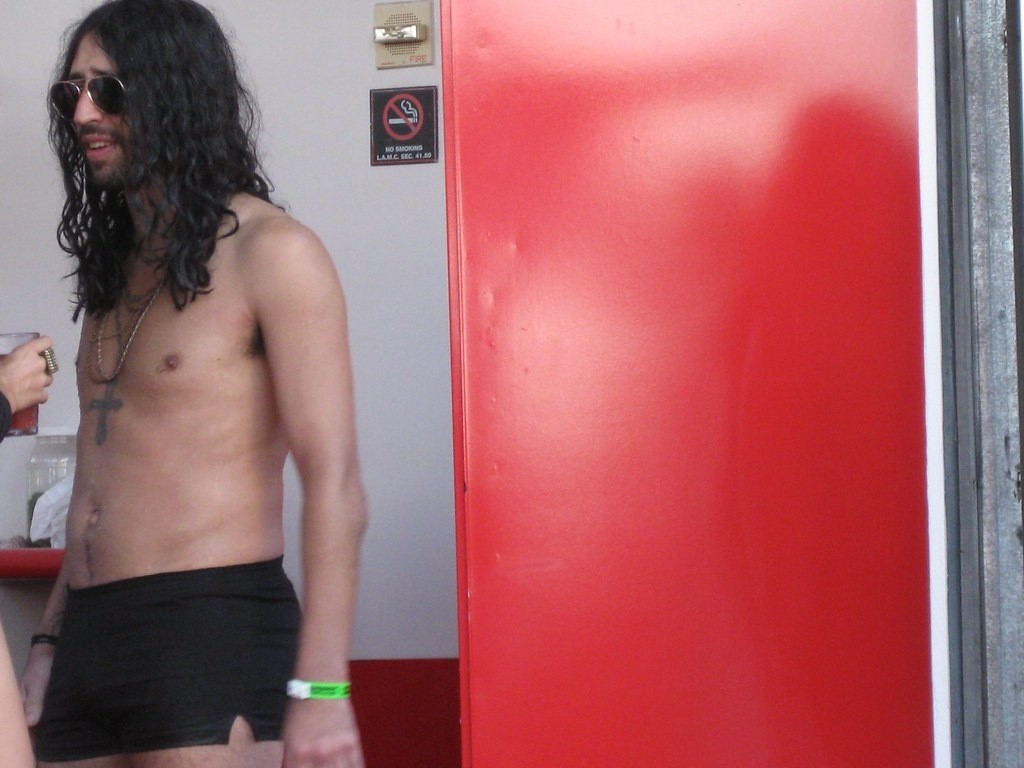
41 348 58 373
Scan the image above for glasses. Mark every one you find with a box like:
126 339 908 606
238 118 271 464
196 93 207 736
49 75 127 121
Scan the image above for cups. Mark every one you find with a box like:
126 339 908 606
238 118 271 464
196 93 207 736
0 330 44 437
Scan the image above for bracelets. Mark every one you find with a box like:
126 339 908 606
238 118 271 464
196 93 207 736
31 635 57 647
284 679 353 704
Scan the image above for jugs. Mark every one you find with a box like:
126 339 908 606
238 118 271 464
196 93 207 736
26 433 78 550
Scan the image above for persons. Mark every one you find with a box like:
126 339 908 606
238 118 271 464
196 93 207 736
0 335 57 768
20 0 370 768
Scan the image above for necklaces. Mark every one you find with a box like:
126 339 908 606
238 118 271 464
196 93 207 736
94 280 168 381
